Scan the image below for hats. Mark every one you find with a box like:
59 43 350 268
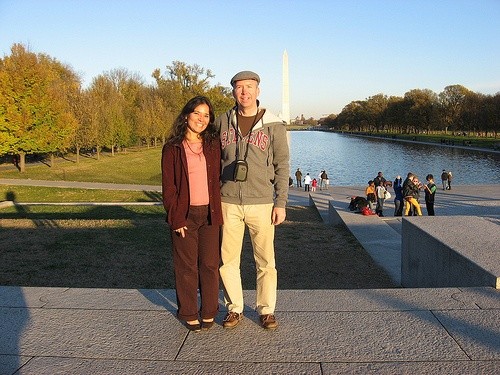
231 71 260 87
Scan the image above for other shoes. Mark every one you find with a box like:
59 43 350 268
200 317 215 332
183 319 201 332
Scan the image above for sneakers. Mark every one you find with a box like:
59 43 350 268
223 312 243 328
259 313 278 329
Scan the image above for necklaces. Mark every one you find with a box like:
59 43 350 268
185 138 203 155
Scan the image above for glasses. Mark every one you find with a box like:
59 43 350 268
414 181 417 182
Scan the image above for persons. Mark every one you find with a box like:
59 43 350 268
423 174 437 216
403 172 423 216
441 169 452 191
295 168 303 188
304 173 312 192
311 178 317 192
366 172 387 217
217 71 289 330
317 170 329 191
161 96 224 331
393 176 403 217
